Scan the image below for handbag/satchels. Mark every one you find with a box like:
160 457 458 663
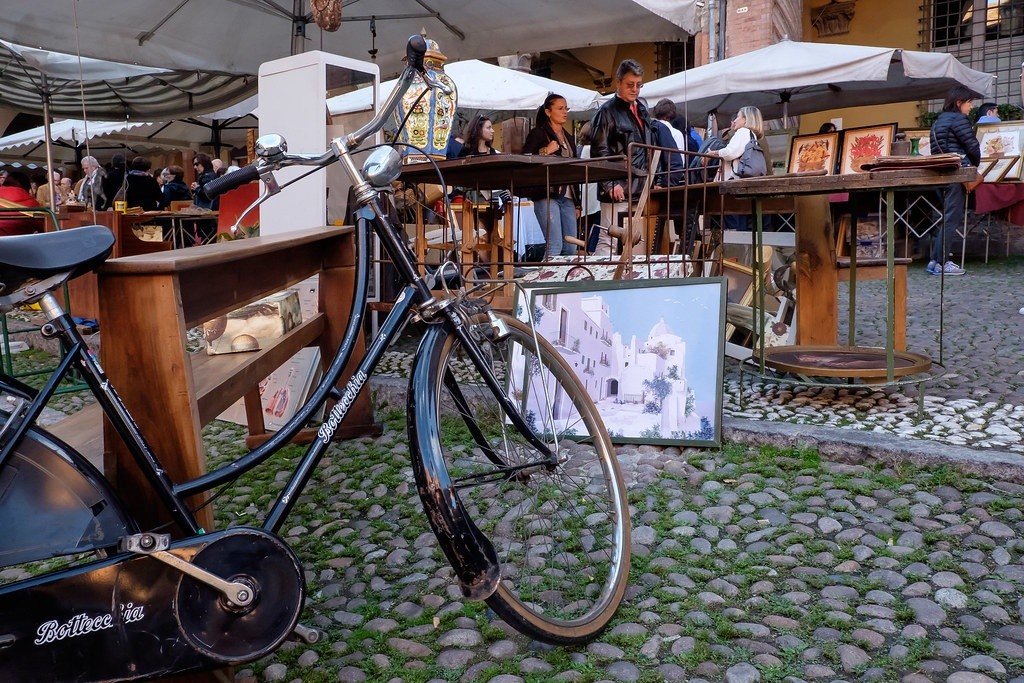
514 185 559 199
963 172 983 194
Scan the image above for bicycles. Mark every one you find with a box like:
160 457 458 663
0 34 634 683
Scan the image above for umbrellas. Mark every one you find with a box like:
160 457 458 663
0 0 696 78
0 93 260 165
326 58 607 157
596 39 999 132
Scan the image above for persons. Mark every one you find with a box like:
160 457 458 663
977 102 1002 124
702 106 772 239
457 94 704 264
590 61 663 256
817 123 836 133
0 156 244 223
927 86 981 276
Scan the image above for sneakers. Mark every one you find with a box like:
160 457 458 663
926 259 936 272
933 260 966 275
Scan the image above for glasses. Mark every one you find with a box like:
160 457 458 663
476 114 488 123
193 162 201 166
620 79 643 89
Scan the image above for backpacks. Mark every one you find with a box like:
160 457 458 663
688 136 727 182
731 130 767 178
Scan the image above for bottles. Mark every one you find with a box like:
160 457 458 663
910 138 921 157
891 133 911 156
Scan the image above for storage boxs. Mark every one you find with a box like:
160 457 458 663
205 292 304 354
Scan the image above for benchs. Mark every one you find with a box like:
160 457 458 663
45 346 273 477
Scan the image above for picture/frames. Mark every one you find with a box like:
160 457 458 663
788 130 842 174
705 259 766 342
839 122 897 178
971 120 1024 180
501 280 603 426
897 125 933 157
514 275 729 448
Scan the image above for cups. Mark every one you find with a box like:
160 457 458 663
115 200 127 214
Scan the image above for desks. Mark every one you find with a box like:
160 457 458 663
393 150 635 312
633 164 975 379
101 225 386 534
876 182 1024 260
48 208 217 318
